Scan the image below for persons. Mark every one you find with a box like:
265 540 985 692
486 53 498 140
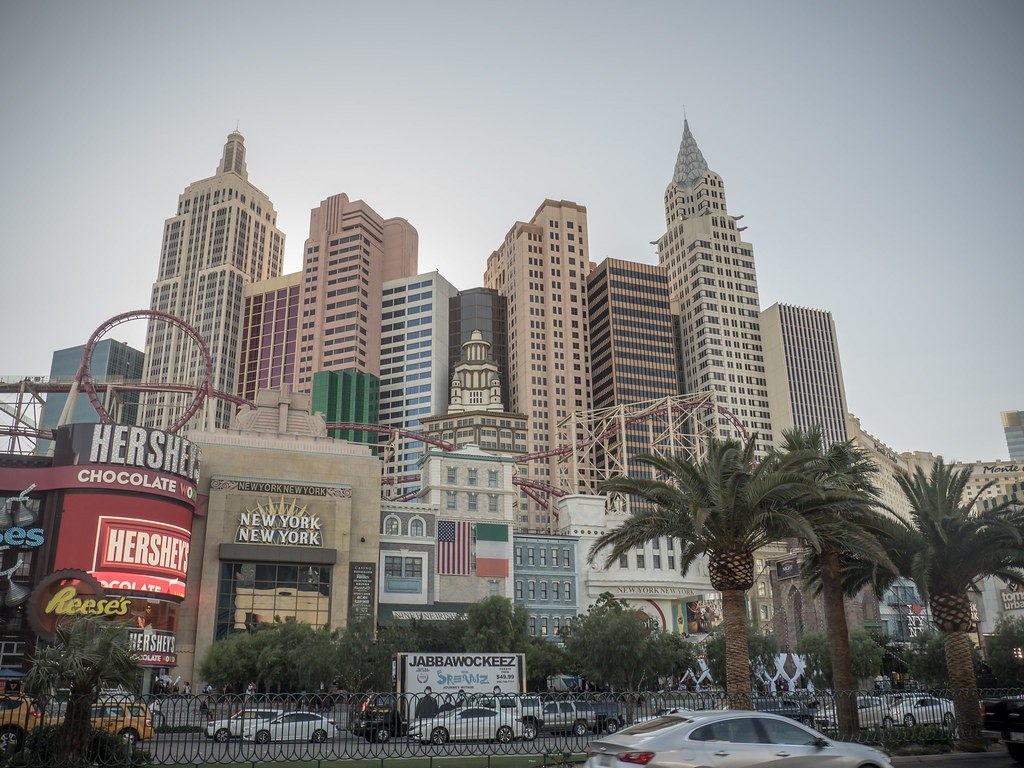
151 678 255 704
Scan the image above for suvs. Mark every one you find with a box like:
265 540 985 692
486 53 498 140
349 689 408 744
47 703 156 743
0 695 50 759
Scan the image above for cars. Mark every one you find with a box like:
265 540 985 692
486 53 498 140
203 707 336 743
471 697 627 742
634 692 1024 729
584 706 895 768
407 707 525 743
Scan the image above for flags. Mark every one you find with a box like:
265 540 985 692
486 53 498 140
437 521 471 575
476 523 509 577
